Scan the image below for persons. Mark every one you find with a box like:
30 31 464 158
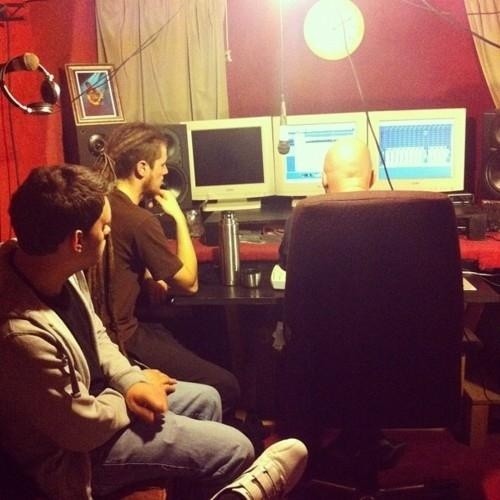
83 122 248 427
277 136 376 273
0 164 256 499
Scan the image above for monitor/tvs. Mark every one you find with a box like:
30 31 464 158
272 112 369 207
367 107 467 193
180 118 275 211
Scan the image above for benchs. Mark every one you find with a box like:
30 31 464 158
94 474 169 500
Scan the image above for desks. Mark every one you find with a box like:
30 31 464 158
176 260 500 430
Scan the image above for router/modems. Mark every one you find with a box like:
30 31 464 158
446 193 474 204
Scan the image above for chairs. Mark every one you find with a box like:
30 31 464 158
256 191 465 499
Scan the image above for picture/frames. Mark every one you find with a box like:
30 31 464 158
64 63 134 127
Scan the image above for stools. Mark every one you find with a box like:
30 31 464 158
454 372 500 452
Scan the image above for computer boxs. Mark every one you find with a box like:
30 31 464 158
202 195 306 246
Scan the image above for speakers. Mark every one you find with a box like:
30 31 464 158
468 211 486 240
68 122 125 175
481 113 500 200
139 123 194 213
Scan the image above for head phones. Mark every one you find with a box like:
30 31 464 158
0 53 61 114
